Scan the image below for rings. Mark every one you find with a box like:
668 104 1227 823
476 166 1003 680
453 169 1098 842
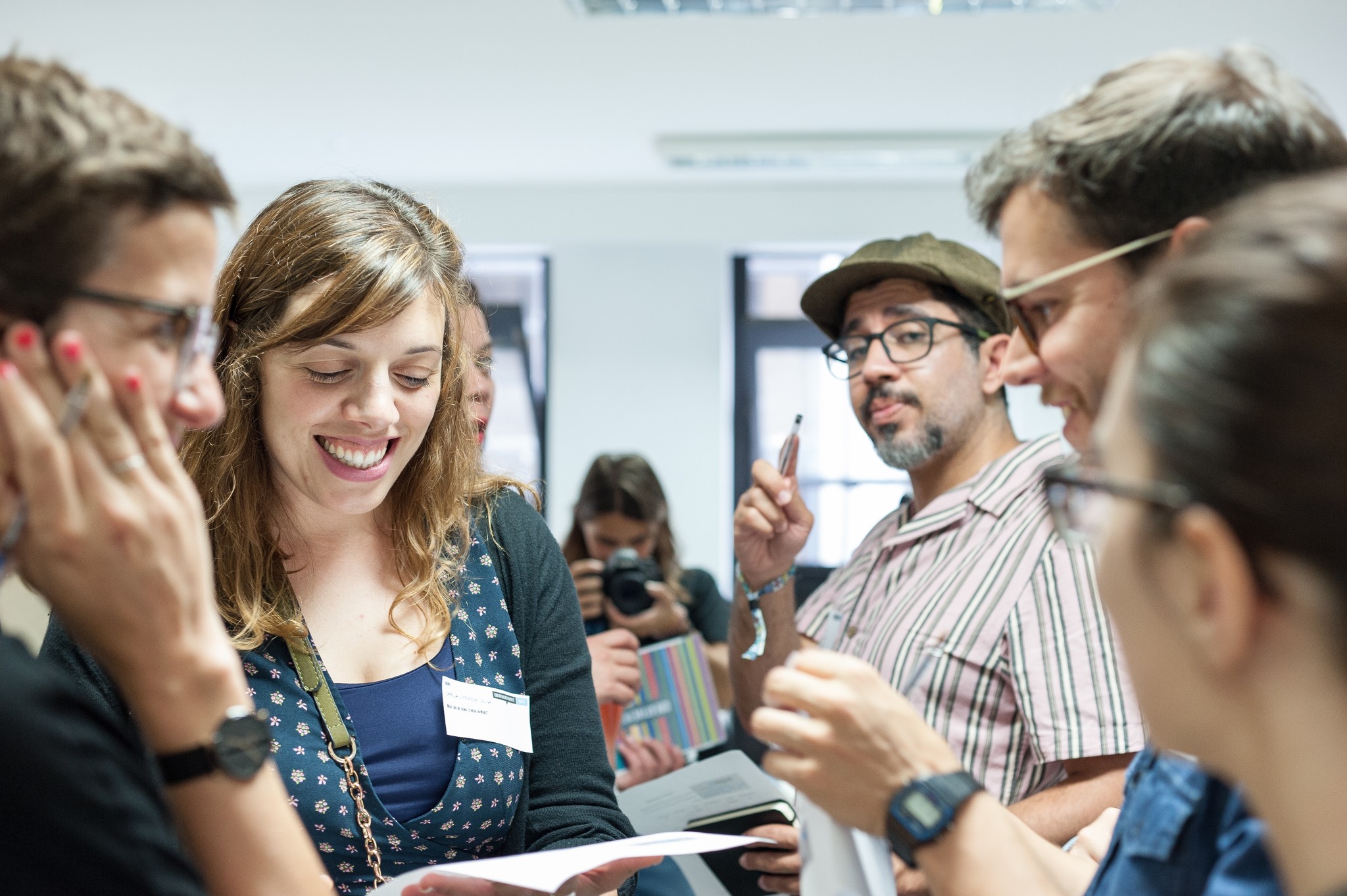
113 452 145 477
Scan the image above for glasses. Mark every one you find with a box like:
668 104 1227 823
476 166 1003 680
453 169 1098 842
824 313 992 379
1002 224 1179 357
75 283 197 367
1042 462 1192 544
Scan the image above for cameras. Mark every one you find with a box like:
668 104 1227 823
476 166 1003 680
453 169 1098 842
603 548 666 616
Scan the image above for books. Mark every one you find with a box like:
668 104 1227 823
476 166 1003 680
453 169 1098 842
684 799 799 896
602 630 724 757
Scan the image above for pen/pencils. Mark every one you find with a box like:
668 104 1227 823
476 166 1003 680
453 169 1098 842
777 415 802 479
1 370 89 559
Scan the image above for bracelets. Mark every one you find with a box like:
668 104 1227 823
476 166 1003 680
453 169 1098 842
736 564 796 662
154 711 278 785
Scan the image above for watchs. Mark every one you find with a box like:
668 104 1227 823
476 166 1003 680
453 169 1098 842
882 770 985 870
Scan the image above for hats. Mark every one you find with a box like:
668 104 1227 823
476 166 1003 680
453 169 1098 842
798 235 1009 335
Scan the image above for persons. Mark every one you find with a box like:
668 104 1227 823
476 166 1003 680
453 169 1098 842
733 233 1144 896
748 53 1347 896
450 275 682 795
560 455 741 710
0 52 342 896
179 182 662 896
1041 170 1347 896
